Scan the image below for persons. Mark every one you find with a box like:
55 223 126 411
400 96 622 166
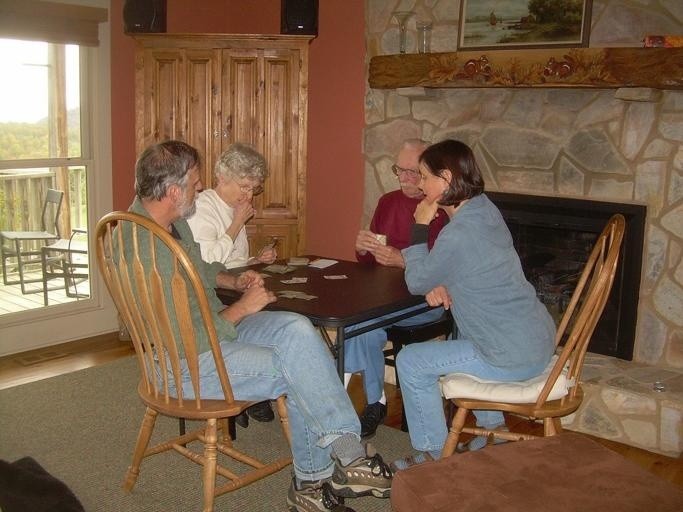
109 140 395 512
389 139 556 475
186 142 278 428
338 138 451 439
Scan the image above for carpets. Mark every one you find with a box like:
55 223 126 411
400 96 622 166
1 350 460 512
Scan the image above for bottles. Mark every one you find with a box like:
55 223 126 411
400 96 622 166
638 35 682 49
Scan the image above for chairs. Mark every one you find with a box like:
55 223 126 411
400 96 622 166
36 224 91 307
0 185 69 293
85 212 296 512
432 210 628 461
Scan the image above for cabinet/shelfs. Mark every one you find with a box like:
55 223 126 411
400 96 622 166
119 27 317 261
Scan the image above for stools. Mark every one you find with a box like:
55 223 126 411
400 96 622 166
363 308 455 397
385 431 682 512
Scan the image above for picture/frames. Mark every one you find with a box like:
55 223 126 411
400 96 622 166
454 0 596 51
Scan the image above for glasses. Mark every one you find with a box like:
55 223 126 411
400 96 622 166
231 177 264 196
287 474 354 512
392 164 418 178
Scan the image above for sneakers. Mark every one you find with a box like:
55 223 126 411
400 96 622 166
389 453 437 474
330 442 393 498
457 427 509 452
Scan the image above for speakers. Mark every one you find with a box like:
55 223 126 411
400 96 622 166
125 0 167 33
280 0 319 35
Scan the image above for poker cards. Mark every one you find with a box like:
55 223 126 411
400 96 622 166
286 257 310 265
277 291 318 300
371 233 386 256
255 243 274 260
280 277 308 285
308 258 339 269
263 264 295 274
260 273 272 279
324 275 348 280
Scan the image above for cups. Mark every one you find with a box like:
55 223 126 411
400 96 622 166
390 10 417 55
414 21 435 54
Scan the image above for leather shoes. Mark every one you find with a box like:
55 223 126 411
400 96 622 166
235 400 273 426
360 400 387 440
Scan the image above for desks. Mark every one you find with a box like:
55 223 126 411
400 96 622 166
214 251 453 410
